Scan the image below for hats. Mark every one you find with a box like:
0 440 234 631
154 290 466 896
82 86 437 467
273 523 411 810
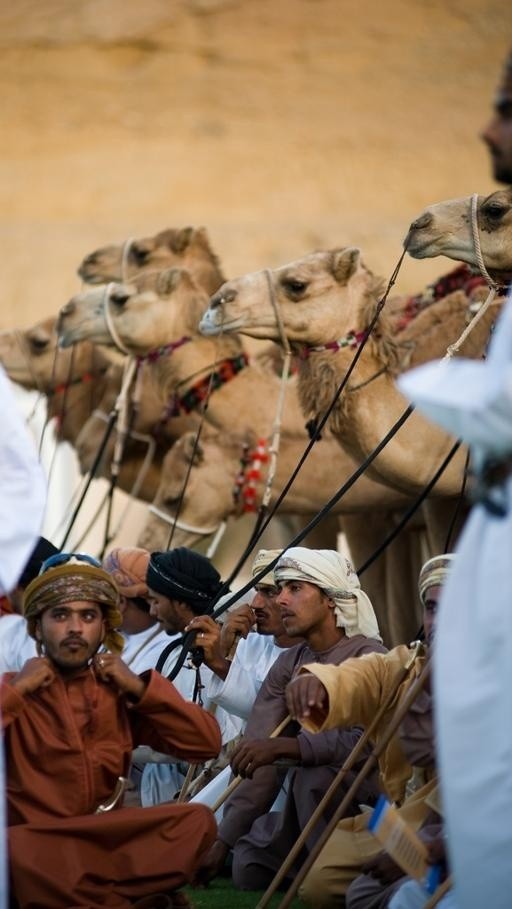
143 545 233 604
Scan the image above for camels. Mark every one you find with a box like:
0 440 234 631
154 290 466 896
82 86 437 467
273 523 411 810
1 227 511 656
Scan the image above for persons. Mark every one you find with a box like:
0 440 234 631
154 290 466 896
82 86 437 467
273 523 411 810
0 544 456 909
